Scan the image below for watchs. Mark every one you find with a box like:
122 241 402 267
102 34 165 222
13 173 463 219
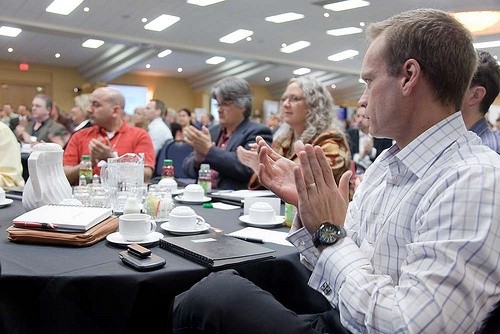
312 222 341 247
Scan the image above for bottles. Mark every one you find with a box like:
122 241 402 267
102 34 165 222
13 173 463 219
78 156 92 186
284 201 298 228
161 160 175 180
197 164 212 194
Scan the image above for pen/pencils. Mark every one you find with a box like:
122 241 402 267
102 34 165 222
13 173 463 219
229 235 264 244
14 222 57 228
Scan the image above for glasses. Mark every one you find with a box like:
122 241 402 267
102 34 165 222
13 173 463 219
280 96 307 103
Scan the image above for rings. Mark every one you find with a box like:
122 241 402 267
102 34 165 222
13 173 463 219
306 183 315 189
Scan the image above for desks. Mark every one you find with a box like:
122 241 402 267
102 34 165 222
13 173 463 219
0 183 331 334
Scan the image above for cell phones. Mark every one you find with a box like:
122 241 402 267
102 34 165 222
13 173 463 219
119 250 166 272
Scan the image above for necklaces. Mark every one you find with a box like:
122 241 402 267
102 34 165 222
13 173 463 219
113 135 120 149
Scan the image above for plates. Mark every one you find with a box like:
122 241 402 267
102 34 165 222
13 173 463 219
106 232 164 248
160 221 209 236
238 215 286 228
0 198 13 208
174 197 213 204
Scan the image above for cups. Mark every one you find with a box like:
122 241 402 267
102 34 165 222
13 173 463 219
76 177 208 239
0 186 6 203
248 202 276 224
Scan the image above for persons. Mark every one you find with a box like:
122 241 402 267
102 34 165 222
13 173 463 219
236 74 354 201
345 105 392 164
183 76 274 189
173 8 500 334
0 87 214 188
461 50 500 154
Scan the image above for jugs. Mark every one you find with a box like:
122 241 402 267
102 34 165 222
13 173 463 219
21 142 72 212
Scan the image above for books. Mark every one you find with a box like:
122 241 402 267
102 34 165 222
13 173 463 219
159 232 276 269
14 204 112 231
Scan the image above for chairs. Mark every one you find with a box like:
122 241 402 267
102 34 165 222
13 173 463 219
154 139 197 186
